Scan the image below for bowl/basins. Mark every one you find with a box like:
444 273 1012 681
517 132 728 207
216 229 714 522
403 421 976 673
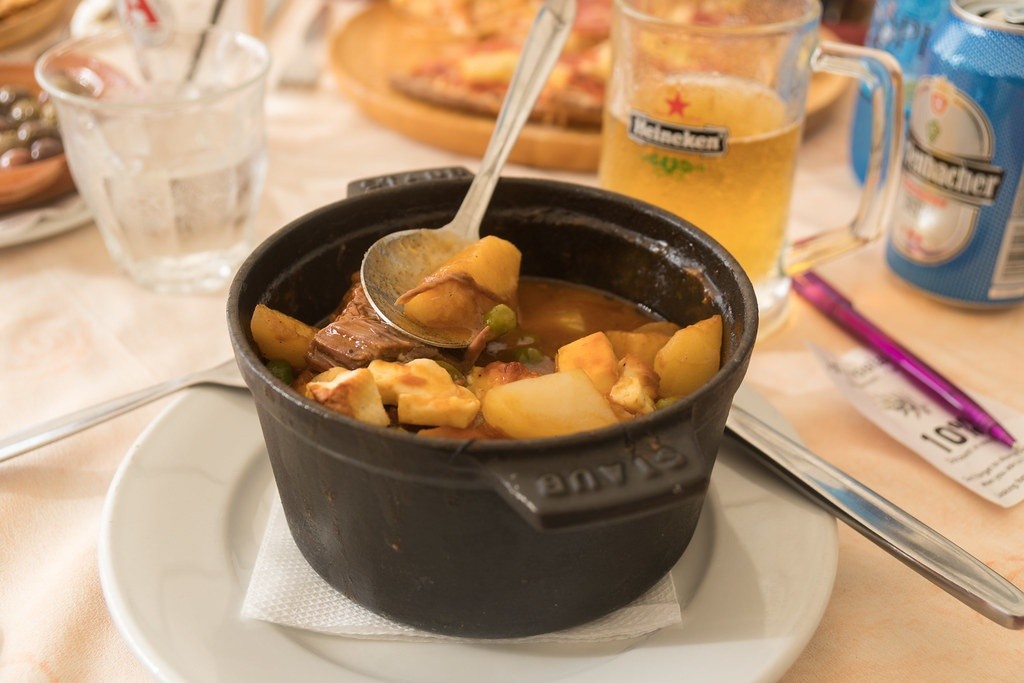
71 0 353 136
226 166 760 640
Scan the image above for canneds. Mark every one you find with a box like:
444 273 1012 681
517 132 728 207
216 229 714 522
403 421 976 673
846 0 1024 310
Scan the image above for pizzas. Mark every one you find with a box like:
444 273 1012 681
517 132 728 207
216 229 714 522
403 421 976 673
389 0 782 125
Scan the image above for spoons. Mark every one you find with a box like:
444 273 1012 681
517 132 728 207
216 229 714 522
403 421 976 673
361 0 578 351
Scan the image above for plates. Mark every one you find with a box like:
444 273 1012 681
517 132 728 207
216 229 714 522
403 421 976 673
0 191 94 249
99 386 840 683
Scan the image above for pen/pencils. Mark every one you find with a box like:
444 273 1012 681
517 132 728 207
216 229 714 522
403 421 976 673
791 268 1020 448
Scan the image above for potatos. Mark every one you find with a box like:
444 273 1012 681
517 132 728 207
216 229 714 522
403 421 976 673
249 234 724 438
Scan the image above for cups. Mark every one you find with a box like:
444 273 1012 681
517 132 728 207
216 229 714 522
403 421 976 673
600 0 907 340
34 20 273 296
